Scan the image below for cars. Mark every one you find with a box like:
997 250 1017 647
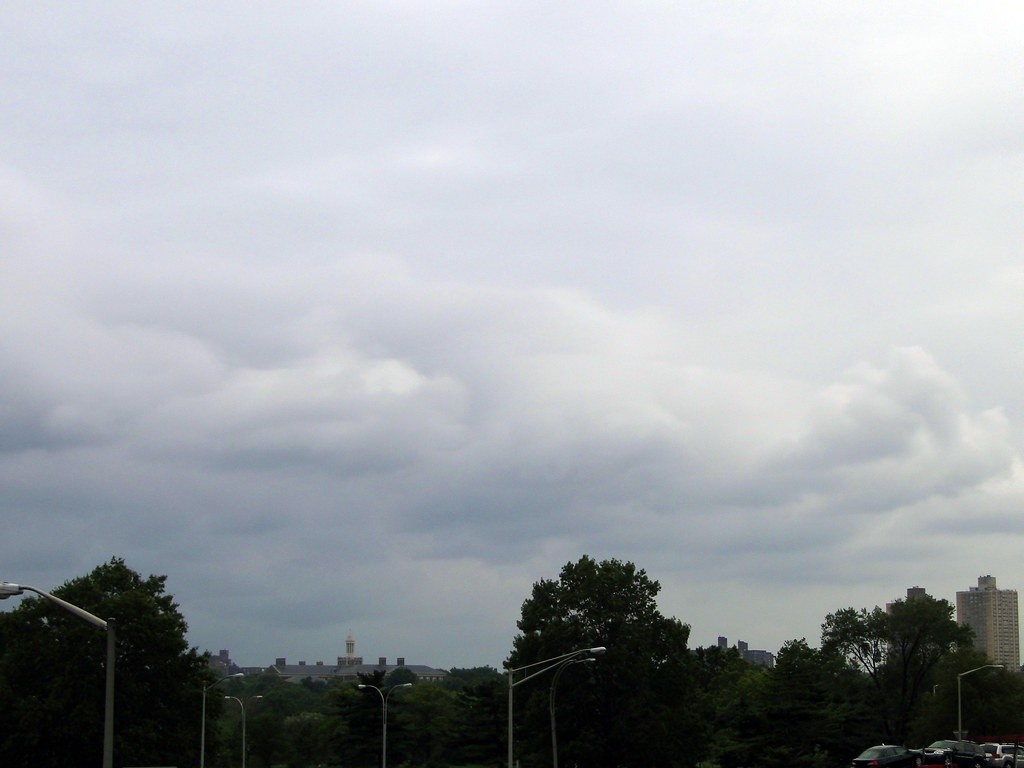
852 738 1024 768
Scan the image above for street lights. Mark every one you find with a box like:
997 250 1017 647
957 664 1005 742
549 656 597 768
506 646 608 768
199 672 244 768
357 682 412 768
223 694 263 768
1 581 116 766
